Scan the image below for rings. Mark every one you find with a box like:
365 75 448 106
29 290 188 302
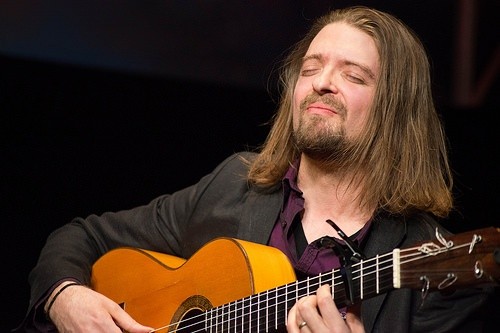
299 321 307 328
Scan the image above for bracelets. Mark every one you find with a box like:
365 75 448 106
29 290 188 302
46 282 82 317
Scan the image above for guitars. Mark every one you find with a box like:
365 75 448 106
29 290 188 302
90 225 500 333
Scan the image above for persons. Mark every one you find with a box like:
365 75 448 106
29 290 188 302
23 8 500 333
